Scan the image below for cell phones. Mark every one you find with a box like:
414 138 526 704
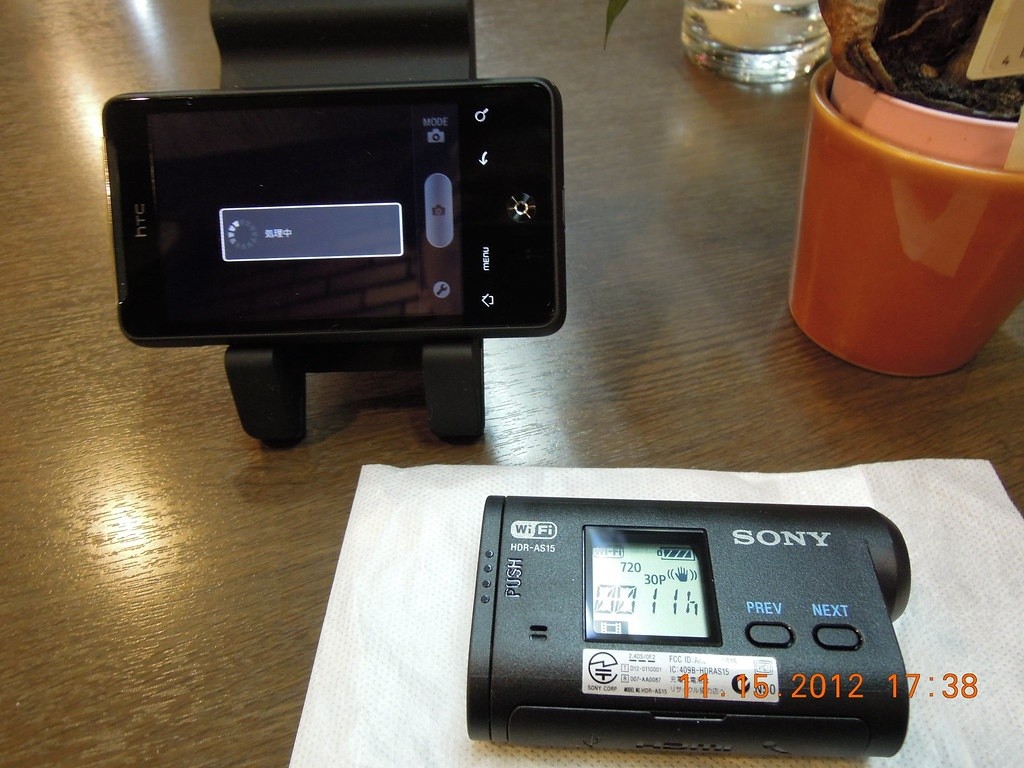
103 75 568 347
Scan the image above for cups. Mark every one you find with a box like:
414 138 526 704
831 71 1015 169
785 62 1024 380
683 1 830 85
